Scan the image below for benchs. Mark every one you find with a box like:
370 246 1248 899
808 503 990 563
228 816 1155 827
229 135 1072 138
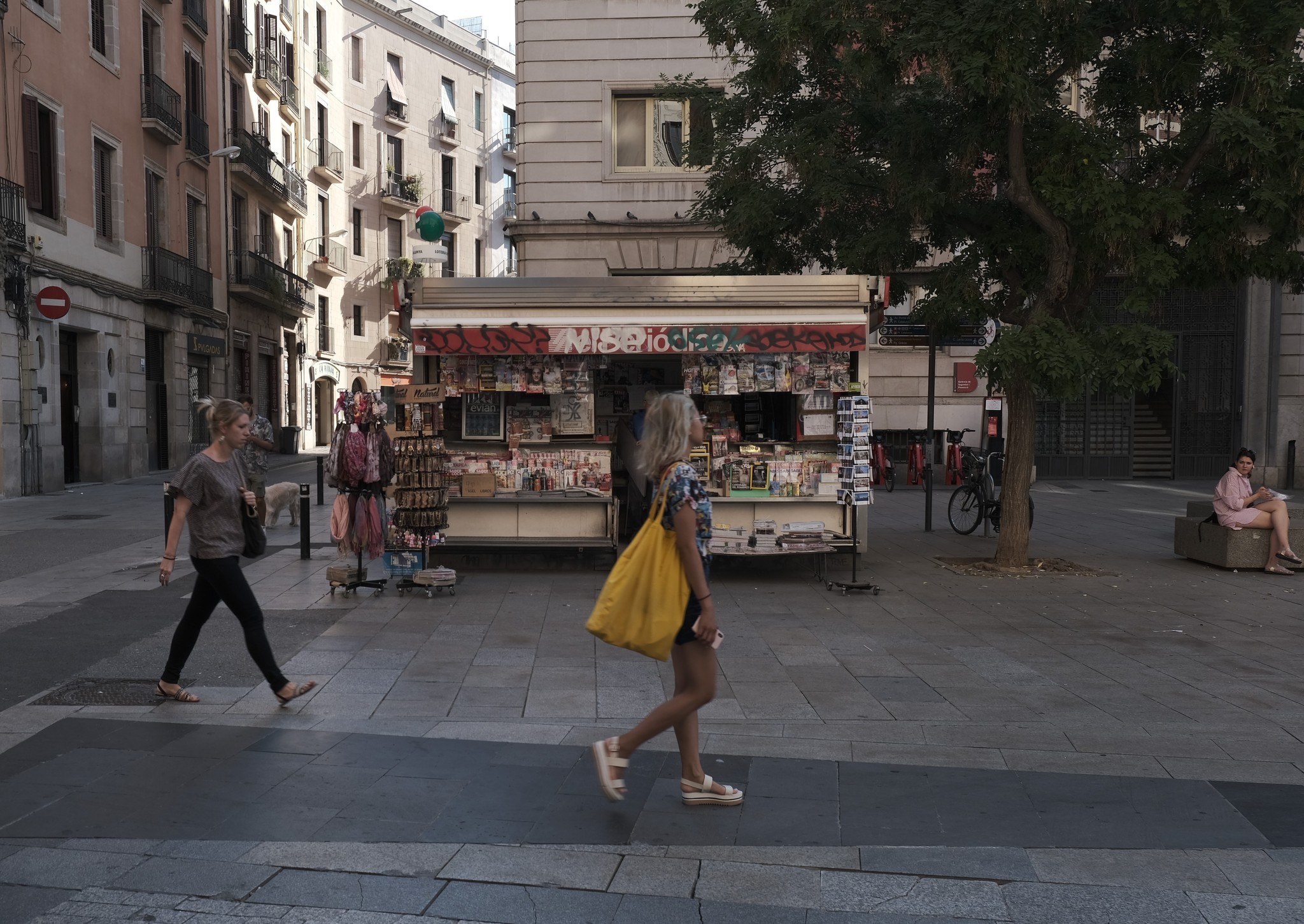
1173 501 1304 569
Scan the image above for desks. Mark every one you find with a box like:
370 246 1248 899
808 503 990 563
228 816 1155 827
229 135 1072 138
708 545 837 591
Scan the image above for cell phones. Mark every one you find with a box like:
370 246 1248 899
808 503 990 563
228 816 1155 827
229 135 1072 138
691 615 725 649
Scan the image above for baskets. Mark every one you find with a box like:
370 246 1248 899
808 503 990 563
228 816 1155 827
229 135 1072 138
960 446 990 469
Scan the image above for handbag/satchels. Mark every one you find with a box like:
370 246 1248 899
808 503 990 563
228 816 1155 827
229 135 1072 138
585 461 693 662
232 448 267 559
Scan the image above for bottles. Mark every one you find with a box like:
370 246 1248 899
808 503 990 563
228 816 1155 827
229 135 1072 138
522 468 554 491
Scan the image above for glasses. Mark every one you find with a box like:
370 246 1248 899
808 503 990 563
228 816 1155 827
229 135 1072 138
691 415 707 423
1239 447 1256 454
246 404 251 411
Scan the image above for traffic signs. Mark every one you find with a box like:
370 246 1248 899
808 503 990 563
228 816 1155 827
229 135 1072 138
879 315 987 347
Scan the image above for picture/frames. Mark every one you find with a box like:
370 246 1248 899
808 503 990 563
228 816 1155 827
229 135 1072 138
744 393 760 432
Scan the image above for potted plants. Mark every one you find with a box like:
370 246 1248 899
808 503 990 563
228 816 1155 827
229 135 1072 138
388 110 397 117
405 171 426 203
387 163 395 179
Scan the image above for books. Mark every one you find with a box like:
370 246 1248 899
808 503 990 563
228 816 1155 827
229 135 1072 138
414 569 456 586
439 355 615 498
683 350 874 555
325 564 368 584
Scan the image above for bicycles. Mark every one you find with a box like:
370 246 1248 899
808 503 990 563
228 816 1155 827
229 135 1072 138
938 428 976 492
948 447 1034 535
902 428 934 492
867 429 898 493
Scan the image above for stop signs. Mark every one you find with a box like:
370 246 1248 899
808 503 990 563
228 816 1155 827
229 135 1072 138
36 286 70 319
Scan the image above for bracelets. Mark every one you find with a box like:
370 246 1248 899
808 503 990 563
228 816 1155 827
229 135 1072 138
162 553 176 560
696 593 712 602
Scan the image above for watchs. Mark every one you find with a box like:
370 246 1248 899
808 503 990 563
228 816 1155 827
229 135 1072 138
392 440 445 527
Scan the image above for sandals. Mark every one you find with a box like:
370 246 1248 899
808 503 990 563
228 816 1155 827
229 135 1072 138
591 735 630 801
680 773 743 806
1275 547 1302 564
155 680 200 702
277 681 319 708
1263 564 1294 576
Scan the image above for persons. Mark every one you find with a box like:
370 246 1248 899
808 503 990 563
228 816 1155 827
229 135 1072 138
236 394 274 540
154 397 319 707
625 389 661 529
1213 447 1302 575
594 391 743 806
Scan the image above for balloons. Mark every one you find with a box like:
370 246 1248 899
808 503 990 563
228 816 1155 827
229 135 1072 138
415 206 445 242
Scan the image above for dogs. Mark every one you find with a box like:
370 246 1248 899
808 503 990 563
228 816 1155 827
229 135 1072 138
263 481 301 529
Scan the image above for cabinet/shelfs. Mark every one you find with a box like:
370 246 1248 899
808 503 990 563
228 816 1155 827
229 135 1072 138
596 360 684 488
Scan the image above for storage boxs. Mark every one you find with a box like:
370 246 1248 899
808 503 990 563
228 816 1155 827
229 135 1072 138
461 473 497 497
815 462 841 494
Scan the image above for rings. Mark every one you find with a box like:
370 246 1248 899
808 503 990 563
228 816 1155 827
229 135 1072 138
161 574 165 578
1267 495 1268 497
